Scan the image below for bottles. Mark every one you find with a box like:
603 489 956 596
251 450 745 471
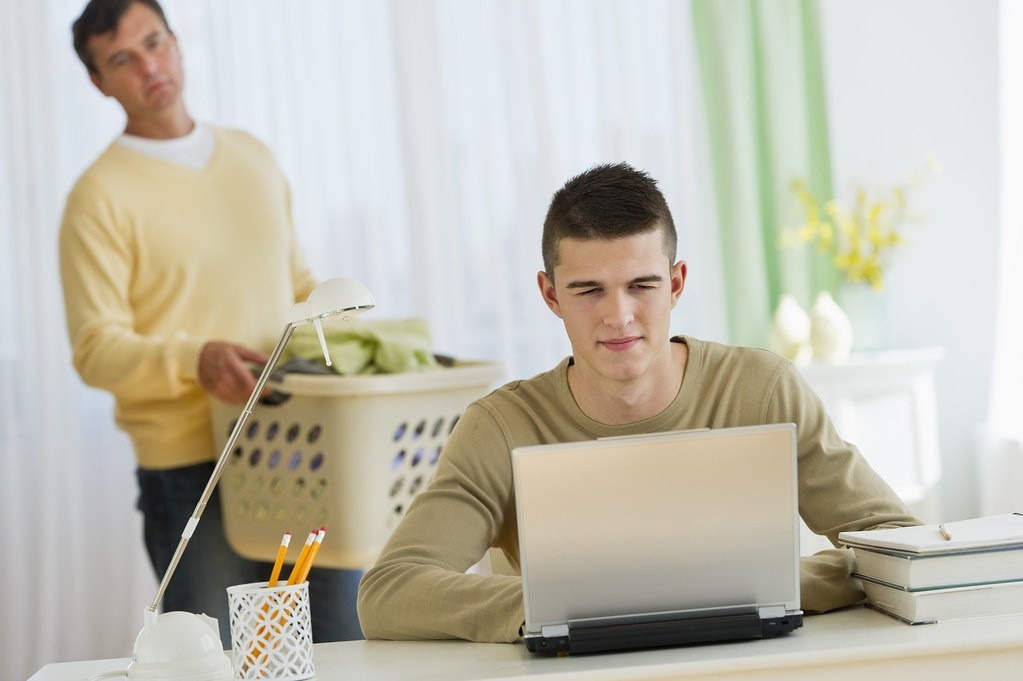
774 293 813 360
811 292 853 351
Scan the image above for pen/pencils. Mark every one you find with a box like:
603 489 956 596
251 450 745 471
236 526 328 680
938 523 951 540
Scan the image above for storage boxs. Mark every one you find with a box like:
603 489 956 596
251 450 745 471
204 357 503 571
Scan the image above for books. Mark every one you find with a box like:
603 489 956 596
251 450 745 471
837 512 1023 627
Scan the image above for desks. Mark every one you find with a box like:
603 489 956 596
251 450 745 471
26 578 1023 681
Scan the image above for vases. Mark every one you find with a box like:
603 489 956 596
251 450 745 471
770 288 850 356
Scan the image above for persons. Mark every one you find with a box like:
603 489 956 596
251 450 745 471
58 0 367 650
356 162 924 643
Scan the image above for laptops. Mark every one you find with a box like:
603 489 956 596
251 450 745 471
512 423 803 656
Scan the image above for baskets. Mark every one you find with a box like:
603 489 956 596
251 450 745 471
211 356 505 575
